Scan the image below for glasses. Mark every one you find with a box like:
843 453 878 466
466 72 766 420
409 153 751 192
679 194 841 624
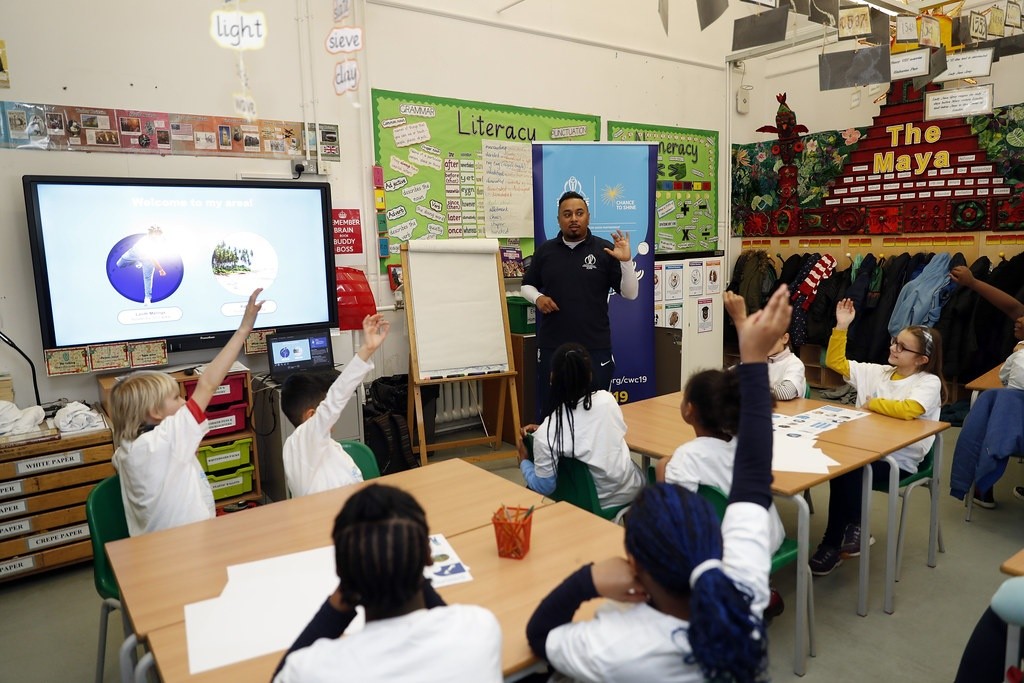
891 336 928 358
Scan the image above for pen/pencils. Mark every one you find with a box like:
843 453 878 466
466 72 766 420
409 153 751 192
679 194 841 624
509 505 534 551
492 501 524 558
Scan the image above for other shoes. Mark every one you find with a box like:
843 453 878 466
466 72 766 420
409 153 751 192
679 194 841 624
1013 486 1024 501
974 486 996 509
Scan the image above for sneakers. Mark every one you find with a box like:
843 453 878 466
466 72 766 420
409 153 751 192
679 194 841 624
842 525 876 558
810 543 842 576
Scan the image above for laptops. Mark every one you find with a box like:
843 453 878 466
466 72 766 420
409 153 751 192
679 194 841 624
265 328 335 387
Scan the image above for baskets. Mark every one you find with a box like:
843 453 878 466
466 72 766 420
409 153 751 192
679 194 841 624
492 507 533 559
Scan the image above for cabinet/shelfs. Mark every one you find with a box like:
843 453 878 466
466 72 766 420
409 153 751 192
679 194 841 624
0 402 115 583
97 359 262 509
250 374 365 503
481 327 682 447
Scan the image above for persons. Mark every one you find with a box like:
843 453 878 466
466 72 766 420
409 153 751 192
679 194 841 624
269 482 504 683
108 288 266 538
808 298 949 575
279 313 390 499
655 367 785 628
948 266 1024 508
518 343 647 509
525 284 793 683
728 332 806 401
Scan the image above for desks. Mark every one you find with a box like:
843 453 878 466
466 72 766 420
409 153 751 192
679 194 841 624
105 459 630 683
618 390 952 677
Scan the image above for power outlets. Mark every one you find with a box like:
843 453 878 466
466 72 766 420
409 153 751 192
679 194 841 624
291 159 318 175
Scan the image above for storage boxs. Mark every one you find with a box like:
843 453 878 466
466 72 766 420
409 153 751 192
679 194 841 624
194 438 253 473
206 464 255 501
506 296 536 334
204 403 247 437
184 373 245 406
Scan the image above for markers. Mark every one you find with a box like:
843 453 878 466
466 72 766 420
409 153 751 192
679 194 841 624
447 373 464 378
487 370 500 373
430 376 443 379
468 372 485 375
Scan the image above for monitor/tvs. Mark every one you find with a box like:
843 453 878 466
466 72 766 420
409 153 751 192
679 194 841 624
22 175 339 353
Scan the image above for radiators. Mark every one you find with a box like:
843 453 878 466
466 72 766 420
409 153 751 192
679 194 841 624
434 291 521 425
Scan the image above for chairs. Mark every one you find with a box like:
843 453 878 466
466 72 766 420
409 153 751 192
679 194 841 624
87 471 132 683
524 433 656 521
336 439 382 482
872 445 946 582
692 483 817 658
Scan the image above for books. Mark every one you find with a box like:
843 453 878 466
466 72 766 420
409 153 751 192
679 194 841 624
520 191 639 425
500 249 524 278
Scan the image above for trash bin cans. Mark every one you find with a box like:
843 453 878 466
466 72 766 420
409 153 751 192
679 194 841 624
373 373 439 458
